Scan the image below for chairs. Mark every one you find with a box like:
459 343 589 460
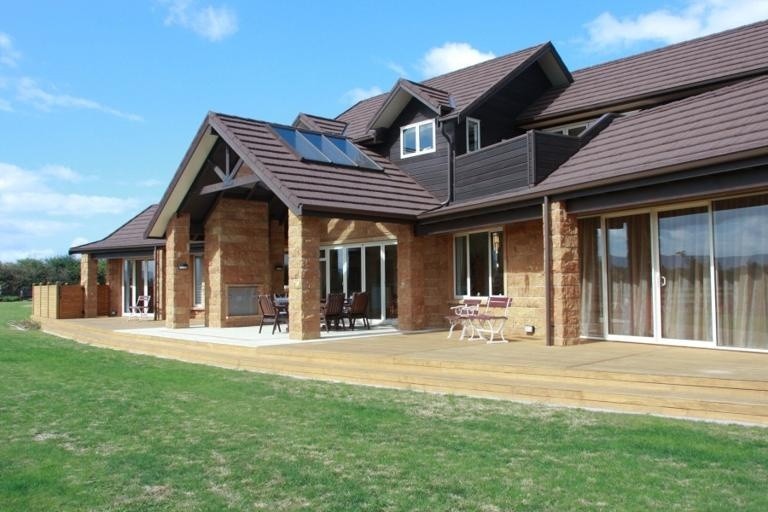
128 295 151 320
445 294 513 346
256 291 370 335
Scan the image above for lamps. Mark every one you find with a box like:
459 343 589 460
178 263 188 270
